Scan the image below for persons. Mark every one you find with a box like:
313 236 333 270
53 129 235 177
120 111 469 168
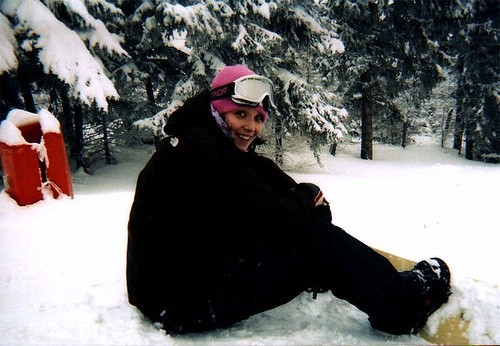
126 65 450 336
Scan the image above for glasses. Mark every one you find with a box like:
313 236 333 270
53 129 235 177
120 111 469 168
210 73 277 116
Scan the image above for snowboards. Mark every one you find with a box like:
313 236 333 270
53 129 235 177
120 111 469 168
366 247 500 345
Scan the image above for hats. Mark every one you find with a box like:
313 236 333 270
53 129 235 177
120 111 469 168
210 64 269 121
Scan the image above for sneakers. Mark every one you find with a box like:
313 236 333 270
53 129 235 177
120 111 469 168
368 259 450 334
